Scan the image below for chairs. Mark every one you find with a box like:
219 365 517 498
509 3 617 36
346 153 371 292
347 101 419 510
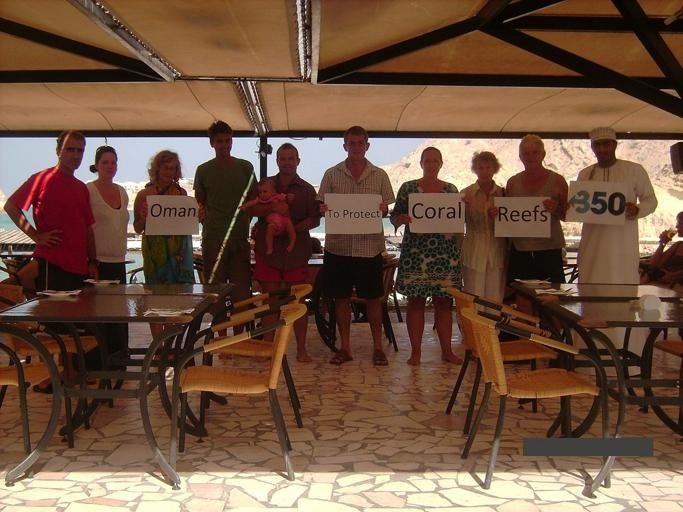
2 249 402 486
442 280 683 490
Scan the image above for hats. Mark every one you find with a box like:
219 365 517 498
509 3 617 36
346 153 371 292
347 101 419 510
588 126 617 141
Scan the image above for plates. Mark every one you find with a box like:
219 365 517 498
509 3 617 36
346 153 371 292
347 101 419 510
84 278 122 287
142 307 196 319
534 288 575 296
515 279 553 285
39 296 81 302
36 289 82 297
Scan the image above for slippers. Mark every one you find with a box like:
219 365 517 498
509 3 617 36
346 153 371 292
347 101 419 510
33 381 64 394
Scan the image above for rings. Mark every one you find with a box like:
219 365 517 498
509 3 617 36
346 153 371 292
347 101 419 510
550 206 553 209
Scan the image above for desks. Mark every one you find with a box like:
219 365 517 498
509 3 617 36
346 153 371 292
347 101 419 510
561 251 579 282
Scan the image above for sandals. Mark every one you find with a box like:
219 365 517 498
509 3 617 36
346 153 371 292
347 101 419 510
372 349 389 366
441 352 463 365
407 354 421 366
329 349 354 366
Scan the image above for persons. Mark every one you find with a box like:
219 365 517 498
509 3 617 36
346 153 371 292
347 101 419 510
4 131 99 395
639 212 683 283
488 135 569 331
85 146 130 371
460 151 507 343
239 177 296 256
192 122 259 359
315 126 396 366
390 147 464 365
563 126 658 378
246 143 321 363
133 149 204 363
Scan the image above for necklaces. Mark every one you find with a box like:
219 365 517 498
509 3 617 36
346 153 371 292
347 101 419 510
155 185 170 196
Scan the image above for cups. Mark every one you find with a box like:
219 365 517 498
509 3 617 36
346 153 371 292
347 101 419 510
658 228 678 247
